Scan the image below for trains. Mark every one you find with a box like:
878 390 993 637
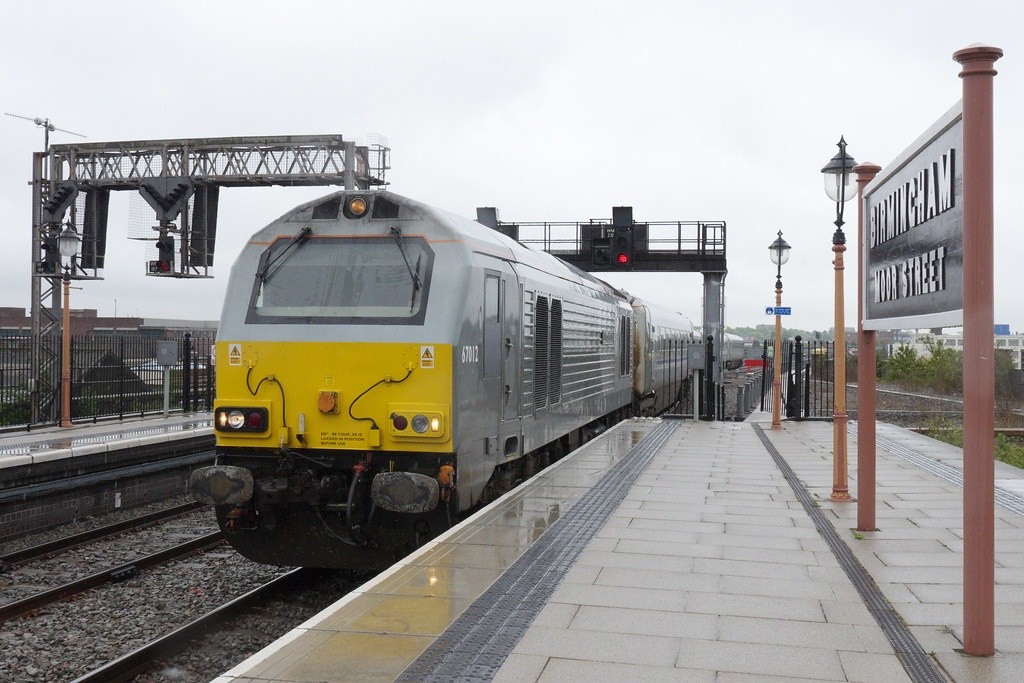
189 189 746 568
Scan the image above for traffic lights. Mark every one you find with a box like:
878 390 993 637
155 236 175 275
612 228 633 267
42 180 79 220
139 177 193 221
40 238 57 273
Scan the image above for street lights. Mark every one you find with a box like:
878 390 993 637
821 134 859 501
769 228 792 431
